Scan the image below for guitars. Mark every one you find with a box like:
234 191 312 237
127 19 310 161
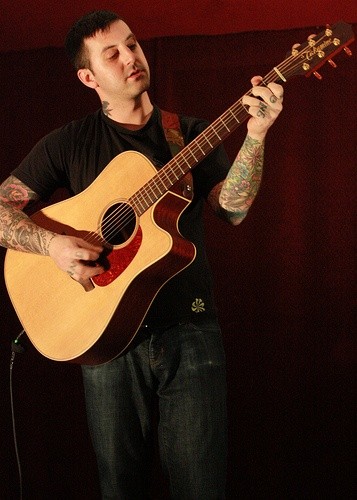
4 24 355 365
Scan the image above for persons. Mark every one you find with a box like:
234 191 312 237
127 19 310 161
1 10 284 500
